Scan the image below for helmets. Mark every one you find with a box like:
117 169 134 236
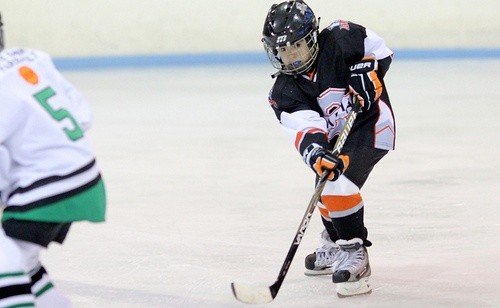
262 0 321 79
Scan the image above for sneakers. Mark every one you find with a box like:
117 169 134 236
304 229 333 275
332 239 372 298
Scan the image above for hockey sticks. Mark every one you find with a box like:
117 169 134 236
230 99 360 305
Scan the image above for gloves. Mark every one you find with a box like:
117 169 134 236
294 128 349 182
350 57 382 112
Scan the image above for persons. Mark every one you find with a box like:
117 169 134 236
261 0 396 298
0 10 106 308
